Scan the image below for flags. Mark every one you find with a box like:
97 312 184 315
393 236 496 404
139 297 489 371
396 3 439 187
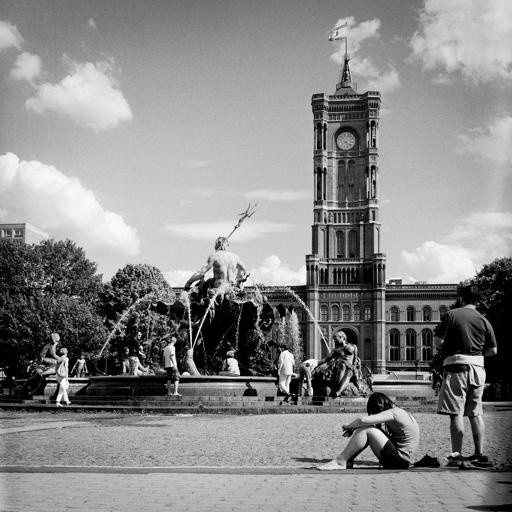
328 23 347 42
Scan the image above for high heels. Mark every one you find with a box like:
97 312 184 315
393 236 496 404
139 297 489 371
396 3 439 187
55 400 72 408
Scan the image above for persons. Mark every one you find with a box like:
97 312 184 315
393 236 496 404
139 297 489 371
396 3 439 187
129 331 147 370
219 351 240 377
162 336 183 396
183 237 249 295
120 346 149 376
51 341 72 407
297 359 318 396
317 392 419 470
71 352 89 378
278 347 294 403
318 331 357 397
433 292 498 461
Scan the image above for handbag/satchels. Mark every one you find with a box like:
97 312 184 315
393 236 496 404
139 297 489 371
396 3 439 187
55 366 67 378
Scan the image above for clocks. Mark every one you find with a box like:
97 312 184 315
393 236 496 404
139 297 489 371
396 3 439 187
337 132 356 151
